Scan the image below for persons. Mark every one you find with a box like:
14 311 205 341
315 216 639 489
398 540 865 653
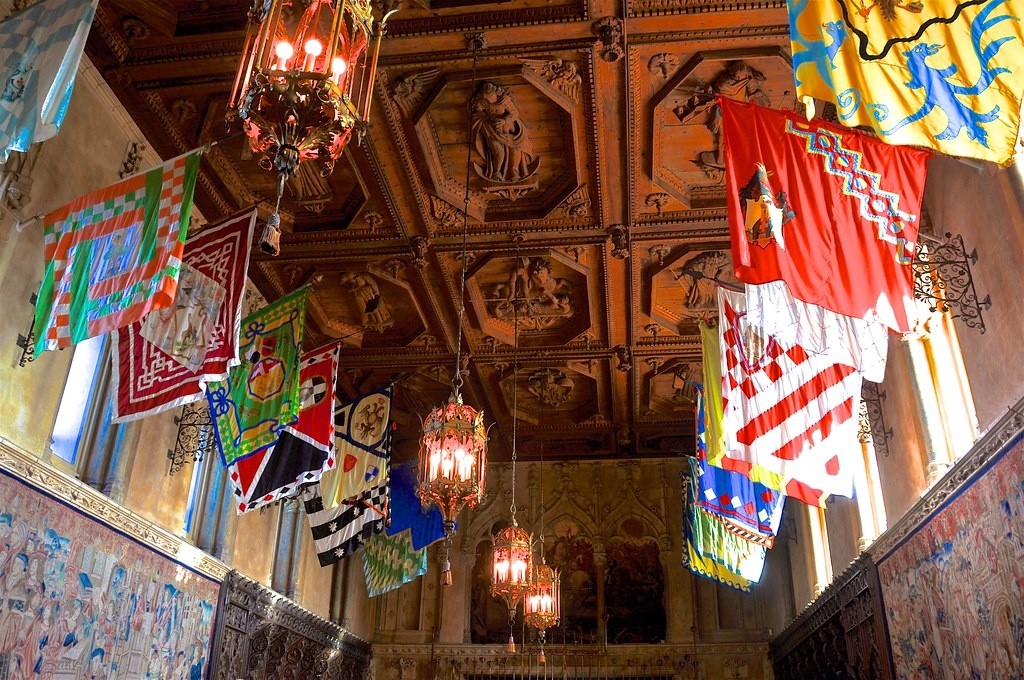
692 62 765 162
466 84 534 183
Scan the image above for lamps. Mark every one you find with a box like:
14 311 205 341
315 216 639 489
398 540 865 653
490 231 538 653
224 0 403 255
413 30 496 586
523 349 564 663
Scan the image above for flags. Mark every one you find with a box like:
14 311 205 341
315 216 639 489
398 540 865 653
0 0 459 600
680 1 1024 594
